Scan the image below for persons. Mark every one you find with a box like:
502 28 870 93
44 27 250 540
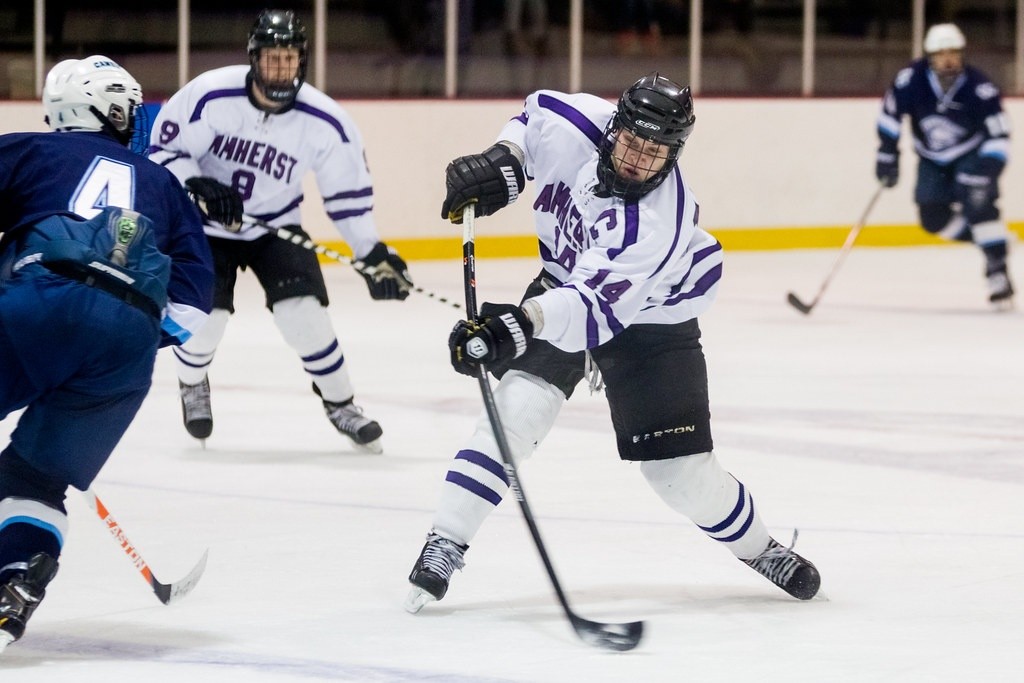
148 9 415 455
0 53 214 653
876 24 1014 309
403 73 834 615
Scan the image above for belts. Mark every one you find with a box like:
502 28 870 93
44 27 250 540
42 258 164 319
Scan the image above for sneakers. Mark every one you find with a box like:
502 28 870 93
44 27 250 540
408 533 469 613
180 381 214 450
326 399 387 454
986 265 1016 309
742 539 828 604
0 552 57 656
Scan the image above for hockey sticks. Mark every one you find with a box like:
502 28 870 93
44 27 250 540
787 148 902 316
459 203 647 653
189 190 466 314
82 487 213 605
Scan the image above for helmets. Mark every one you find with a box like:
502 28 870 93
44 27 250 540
41 55 150 157
247 11 311 101
924 24 965 53
597 73 696 201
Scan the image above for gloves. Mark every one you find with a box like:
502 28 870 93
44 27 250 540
958 154 1002 190
440 144 525 226
874 146 899 188
449 302 535 380
188 176 244 232
354 243 413 300
